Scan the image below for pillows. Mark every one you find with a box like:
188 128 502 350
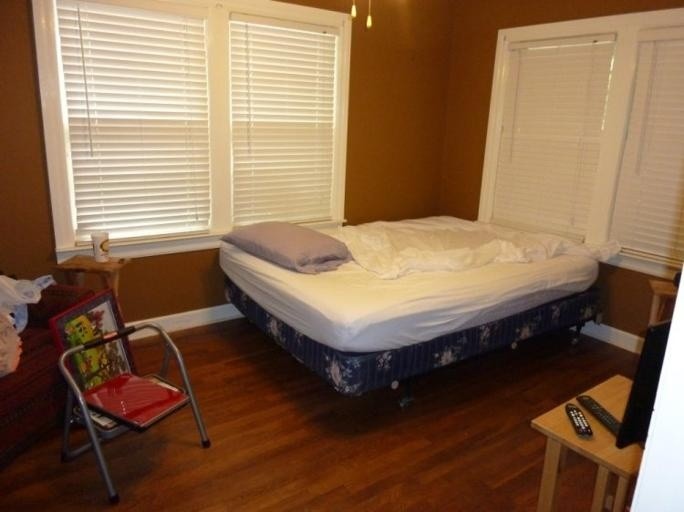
219 220 354 274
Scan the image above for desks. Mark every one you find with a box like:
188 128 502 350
55 254 129 318
648 281 678 326
531 374 644 512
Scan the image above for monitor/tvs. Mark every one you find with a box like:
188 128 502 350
611 320 672 450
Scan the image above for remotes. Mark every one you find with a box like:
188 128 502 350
564 400 592 439
577 392 623 437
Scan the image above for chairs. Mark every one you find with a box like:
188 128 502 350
58 321 210 505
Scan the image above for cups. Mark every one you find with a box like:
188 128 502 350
90 232 110 263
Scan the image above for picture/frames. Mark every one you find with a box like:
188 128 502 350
48 287 138 396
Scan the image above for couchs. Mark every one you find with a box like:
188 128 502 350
0 269 95 473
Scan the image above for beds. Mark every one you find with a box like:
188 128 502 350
219 217 609 417
